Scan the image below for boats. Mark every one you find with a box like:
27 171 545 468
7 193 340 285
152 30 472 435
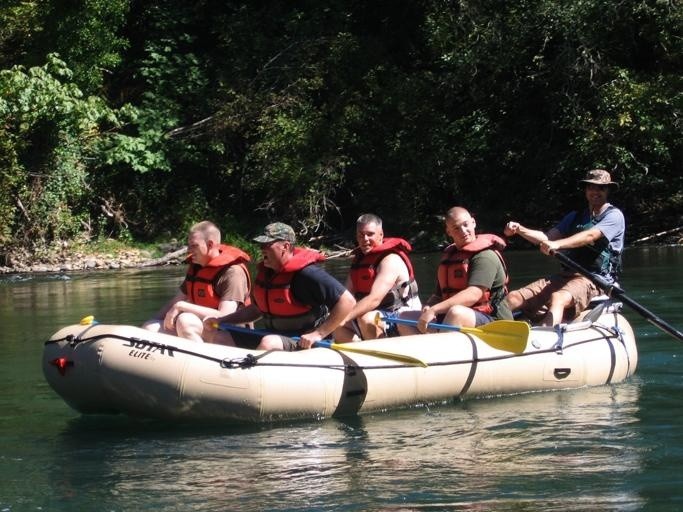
40 304 640 425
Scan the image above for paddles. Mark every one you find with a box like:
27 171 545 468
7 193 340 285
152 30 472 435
382 319 529 354
212 325 426 367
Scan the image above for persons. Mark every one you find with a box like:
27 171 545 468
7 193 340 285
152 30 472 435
396 206 509 334
336 214 423 340
203 221 357 349
141 220 254 343
503 169 626 327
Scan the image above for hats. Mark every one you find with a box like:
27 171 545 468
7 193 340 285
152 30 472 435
251 221 296 243
579 170 619 194
